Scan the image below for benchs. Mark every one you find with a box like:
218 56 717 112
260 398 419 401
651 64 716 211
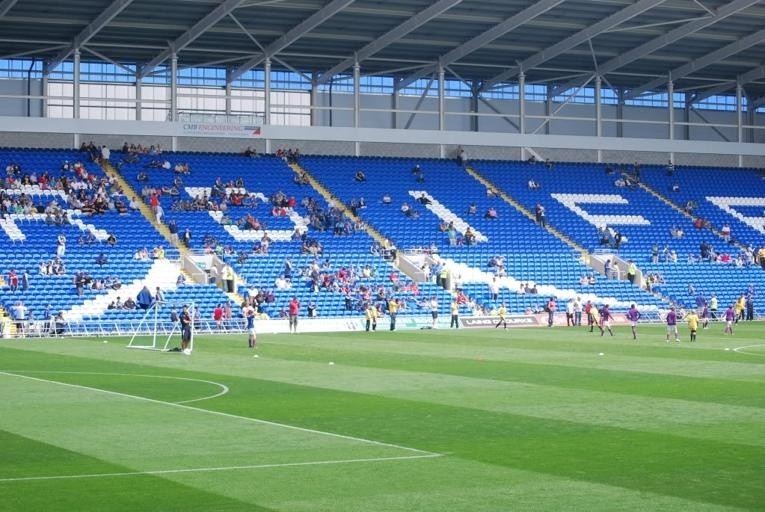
1 146 765 329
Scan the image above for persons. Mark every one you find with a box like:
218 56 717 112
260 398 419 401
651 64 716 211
0 142 764 352
